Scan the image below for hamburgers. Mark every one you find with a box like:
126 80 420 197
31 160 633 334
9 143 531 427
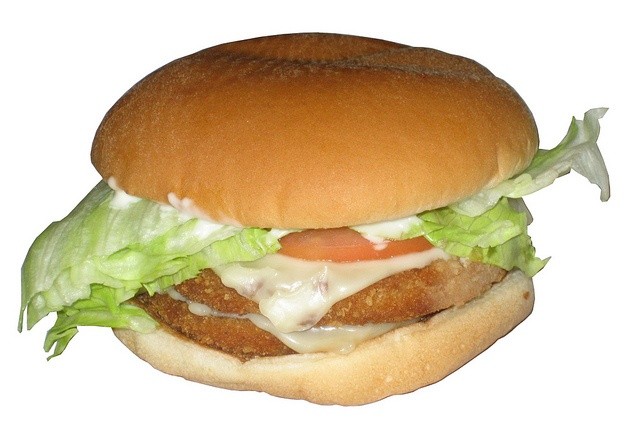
17 32 611 405
